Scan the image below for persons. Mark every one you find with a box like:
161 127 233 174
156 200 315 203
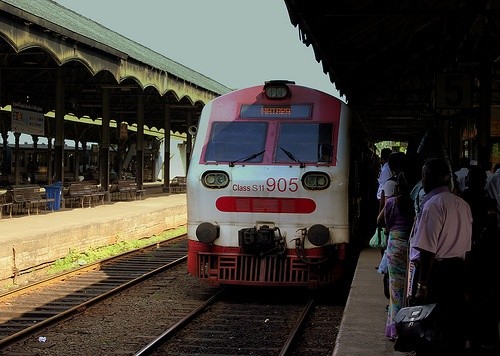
77 162 99 180
353 148 500 356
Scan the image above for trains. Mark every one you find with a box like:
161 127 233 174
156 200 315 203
181 79 358 291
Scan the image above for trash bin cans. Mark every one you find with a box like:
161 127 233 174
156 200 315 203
43 181 63 210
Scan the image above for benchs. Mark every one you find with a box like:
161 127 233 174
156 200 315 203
0 180 147 219
170 176 186 194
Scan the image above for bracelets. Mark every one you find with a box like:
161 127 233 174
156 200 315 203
416 283 426 289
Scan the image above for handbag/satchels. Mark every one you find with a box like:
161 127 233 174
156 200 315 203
392 303 443 349
370 227 389 248
